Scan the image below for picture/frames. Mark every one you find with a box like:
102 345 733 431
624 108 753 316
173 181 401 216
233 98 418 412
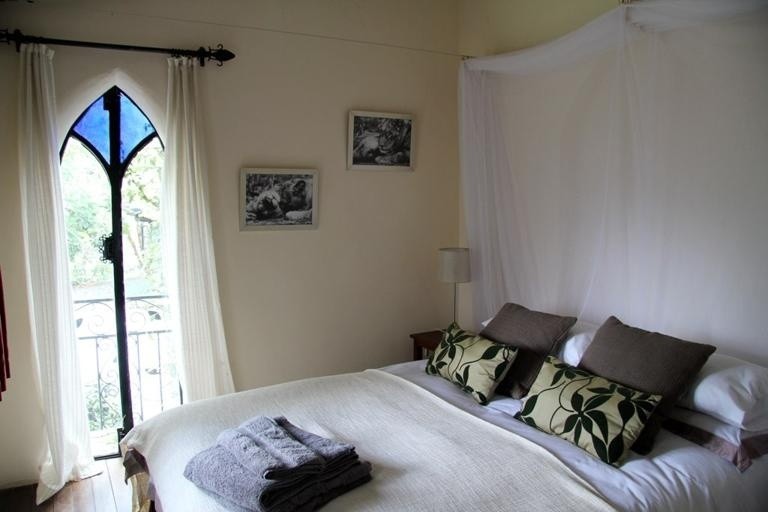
346 110 416 172
239 167 318 230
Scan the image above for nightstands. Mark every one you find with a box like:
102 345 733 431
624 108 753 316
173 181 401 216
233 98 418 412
410 330 442 360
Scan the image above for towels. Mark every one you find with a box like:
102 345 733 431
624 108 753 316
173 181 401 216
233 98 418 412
184 413 374 512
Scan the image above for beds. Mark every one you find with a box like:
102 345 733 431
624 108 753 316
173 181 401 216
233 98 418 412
120 359 768 512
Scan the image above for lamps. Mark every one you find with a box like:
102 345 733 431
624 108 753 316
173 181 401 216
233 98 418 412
439 247 471 323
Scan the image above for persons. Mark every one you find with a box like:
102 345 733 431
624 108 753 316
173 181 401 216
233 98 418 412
255 178 307 220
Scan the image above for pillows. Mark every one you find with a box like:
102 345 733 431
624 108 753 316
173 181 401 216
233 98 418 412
576 316 716 454
555 321 768 431
481 317 493 326
425 322 518 407
479 302 577 399
512 356 665 468
